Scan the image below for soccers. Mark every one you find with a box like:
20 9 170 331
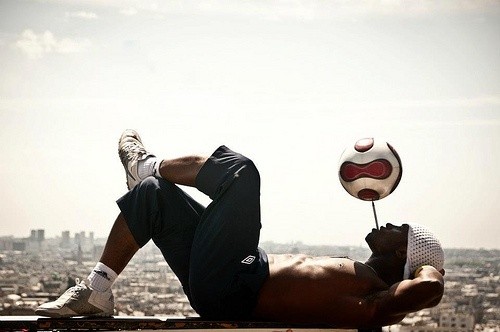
338 137 403 203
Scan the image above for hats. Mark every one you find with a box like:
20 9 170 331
402 222 445 279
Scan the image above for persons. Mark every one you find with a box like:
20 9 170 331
35 129 445 330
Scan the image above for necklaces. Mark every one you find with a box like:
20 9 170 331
330 256 380 279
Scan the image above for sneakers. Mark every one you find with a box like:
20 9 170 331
119 128 157 189
33 276 115 319
